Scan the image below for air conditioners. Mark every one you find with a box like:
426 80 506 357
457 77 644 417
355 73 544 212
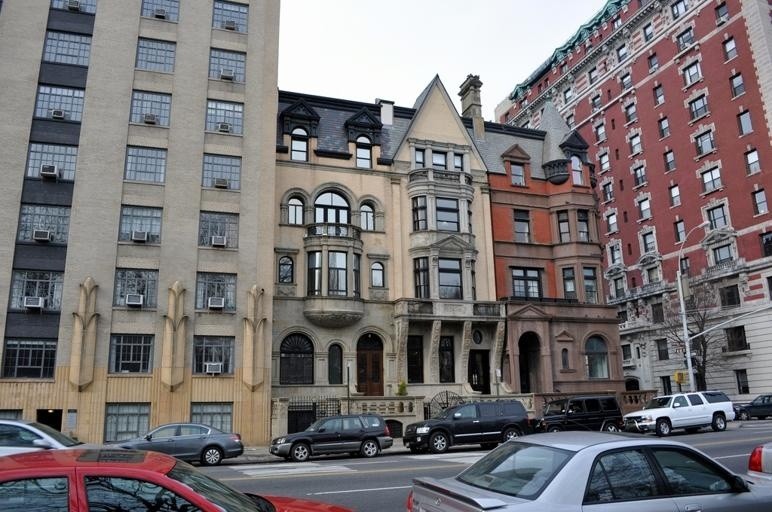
52 108 64 120
68 0 80 11
39 163 60 181
33 229 52 243
125 293 144 306
24 295 46 310
132 229 148 242
204 20 237 380
153 8 166 19
145 113 157 124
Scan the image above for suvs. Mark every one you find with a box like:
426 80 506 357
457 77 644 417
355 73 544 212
269 413 393 462
402 400 530 453
527 396 624 434
623 389 736 437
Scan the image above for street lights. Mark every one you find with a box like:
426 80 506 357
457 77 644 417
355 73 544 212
675 221 712 393
342 359 352 415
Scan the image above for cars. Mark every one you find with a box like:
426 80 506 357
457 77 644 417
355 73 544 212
405 430 771 512
732 394 772 421
102 422 244 465
748 442 771 485
0 449 359 512
1 418 123 457
